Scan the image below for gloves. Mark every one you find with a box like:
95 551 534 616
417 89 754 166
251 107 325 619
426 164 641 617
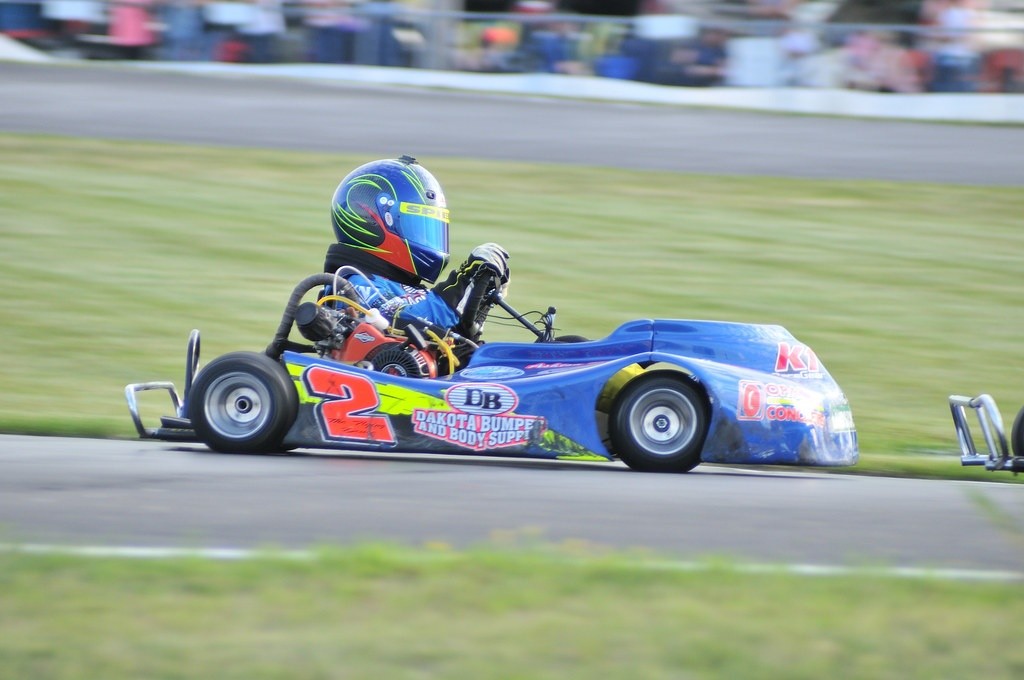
430 243 510 334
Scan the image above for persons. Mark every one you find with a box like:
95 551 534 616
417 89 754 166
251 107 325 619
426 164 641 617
0 0 1024 93
324 155 510 328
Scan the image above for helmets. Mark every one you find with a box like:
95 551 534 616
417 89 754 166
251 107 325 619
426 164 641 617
330 156 450 284
